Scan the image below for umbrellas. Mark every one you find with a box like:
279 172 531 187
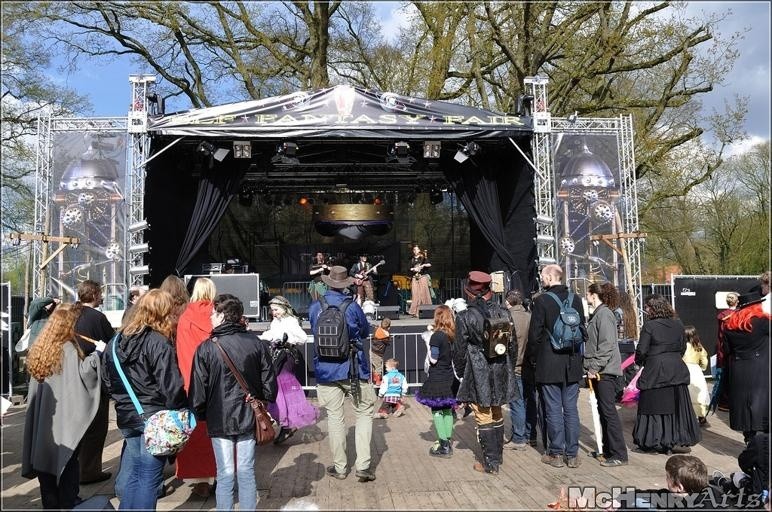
586 371 604 463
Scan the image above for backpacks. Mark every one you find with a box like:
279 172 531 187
543 291 585 355
314 295 359 397
467 304 514 359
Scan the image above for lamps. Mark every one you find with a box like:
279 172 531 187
234 141 253 160
130 263 151 275
538 256 555 263
374 191 386 205
429 186 443 204
128 218 149 234
129 242 150 254
387 142 414 167
537 234 554 243
423 139 441 159
536 215 553 224
272 142 300 170
199 142 229 164
239 191 253 207
455 142 478 162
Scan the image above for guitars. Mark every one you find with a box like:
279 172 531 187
354 260 386 286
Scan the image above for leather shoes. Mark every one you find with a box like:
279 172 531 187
79 472 112 485
273 427 298 444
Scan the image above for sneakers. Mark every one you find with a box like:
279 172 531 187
562 452 582 468
541 454 564 468
193 482 211 497
165 483 175 496
600 456 628 467
526 438 538 447
667 444 691 456
631 446 659 455
717 403 730 412
502 439 527 451
326 465 346 479
463 405 473 417
411 314 417 319
474 463 499 475
355 469 376 481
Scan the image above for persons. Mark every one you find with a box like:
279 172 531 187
502 289 538 452
526 262 587 469
408 244 433 319
444 297 473 425
617 453 742 511
13 251 409 511
709 432 771 511
714 292 738 411
682 326 712 426
451 270 522 475
615 293 638 402
579 281 631 467
631 292 704 454
414 303 459 459
720 289 772 447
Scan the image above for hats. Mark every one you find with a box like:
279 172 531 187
736 291 766 309
29 297 61 321
464 271 493 302
359 251 369 256
738 434 769 492
320 266 354 289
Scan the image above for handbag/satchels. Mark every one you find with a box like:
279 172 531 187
249 399 282 446
144 407 197 457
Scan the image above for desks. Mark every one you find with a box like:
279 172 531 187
184 274 259 322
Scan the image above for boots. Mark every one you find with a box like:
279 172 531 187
393 401 406 418
373 408 389 419
376 374 382 386
433 438 453 455
372 372 377 382
430 438 451 459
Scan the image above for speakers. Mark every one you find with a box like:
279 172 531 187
376 306 400 320
417 305 441 317
